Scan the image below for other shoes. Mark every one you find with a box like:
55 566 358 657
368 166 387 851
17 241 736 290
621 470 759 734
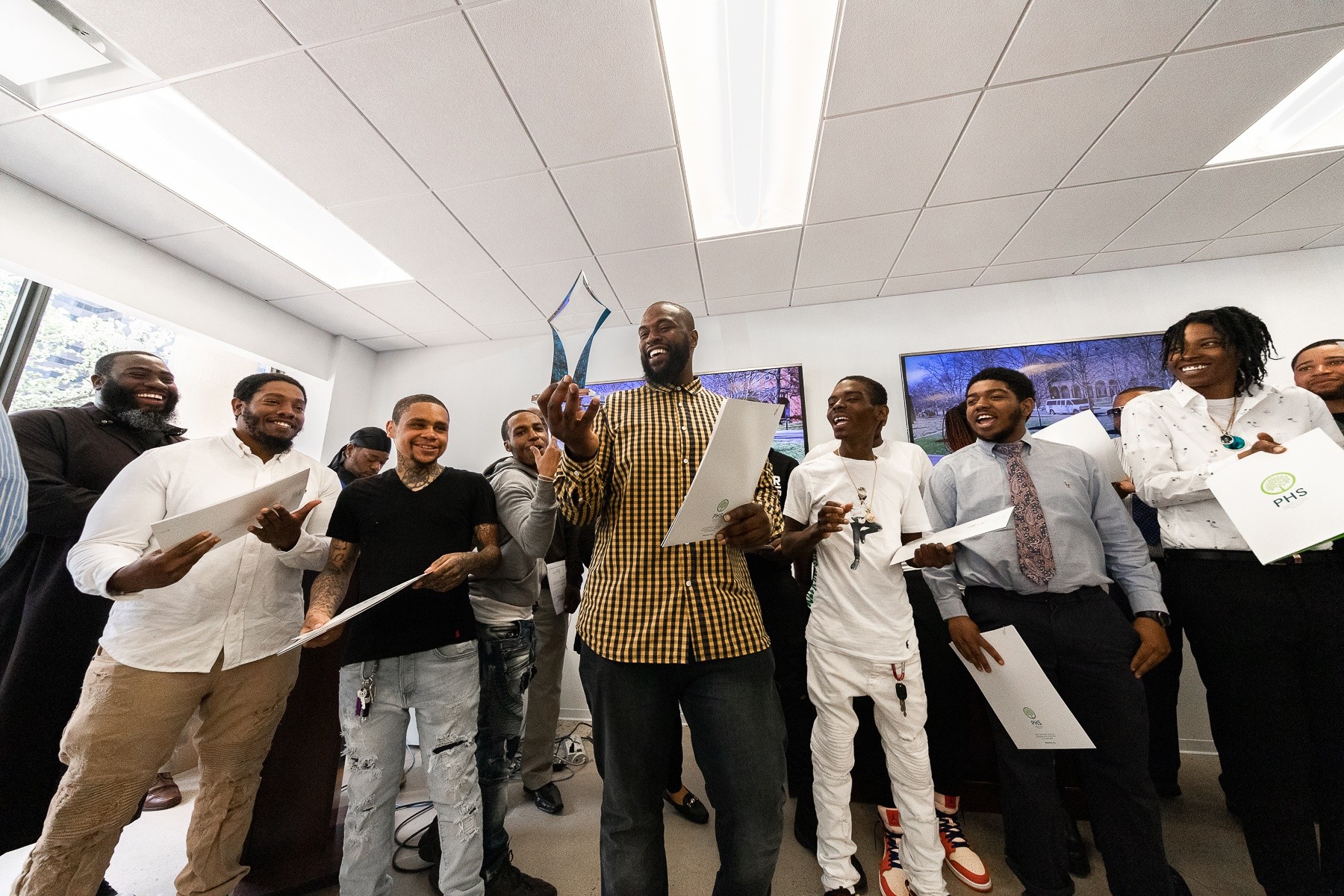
1157 783 1183 799
851 854 868 896
382 874 394 896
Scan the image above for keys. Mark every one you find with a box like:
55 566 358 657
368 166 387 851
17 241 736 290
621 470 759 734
895 683 911 716
355 676 375 724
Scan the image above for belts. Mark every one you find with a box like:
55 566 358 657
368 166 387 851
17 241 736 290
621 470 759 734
1166 549 1316 565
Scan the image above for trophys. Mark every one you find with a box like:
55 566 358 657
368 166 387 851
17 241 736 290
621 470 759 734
530 268 612 405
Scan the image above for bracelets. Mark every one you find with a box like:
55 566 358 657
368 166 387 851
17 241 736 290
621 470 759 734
112 590 144 595
538 474 555 480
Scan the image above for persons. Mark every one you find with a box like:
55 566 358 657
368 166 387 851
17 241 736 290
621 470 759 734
233 426 391 889
941 397 1091 877
10 373 343 896
781 375 959 896
298 393 501 895
1120 305 1343 895
417 410 562 895
920 367 1242 896
509 385 1184 896
0 351 191 896
573 524 709 826
537 301 784 896
1290 337 1342 432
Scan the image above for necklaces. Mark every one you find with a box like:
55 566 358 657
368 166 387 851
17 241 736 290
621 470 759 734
1208 394 1246 451
838 448 877 523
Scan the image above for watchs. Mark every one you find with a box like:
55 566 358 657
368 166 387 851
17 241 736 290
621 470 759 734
1133 610 1173 629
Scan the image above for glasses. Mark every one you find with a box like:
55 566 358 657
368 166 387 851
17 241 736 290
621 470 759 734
1106 406 1124 421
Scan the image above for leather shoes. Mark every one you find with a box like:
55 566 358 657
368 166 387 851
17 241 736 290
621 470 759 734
523 781 563 813
664 784 710 824
142 772 182 812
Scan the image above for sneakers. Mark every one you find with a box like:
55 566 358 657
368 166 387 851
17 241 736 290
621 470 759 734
418 830 442 863
428 860 557 896
877 805 910 896
934 792 992 893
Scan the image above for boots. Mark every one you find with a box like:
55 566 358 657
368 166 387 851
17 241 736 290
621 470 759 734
1061 798 1090 878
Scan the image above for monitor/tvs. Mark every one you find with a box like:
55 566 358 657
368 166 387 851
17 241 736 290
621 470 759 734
551 364 809 468
898 332 1176 466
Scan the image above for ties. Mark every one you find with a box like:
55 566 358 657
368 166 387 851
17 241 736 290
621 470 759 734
994 442 1057 587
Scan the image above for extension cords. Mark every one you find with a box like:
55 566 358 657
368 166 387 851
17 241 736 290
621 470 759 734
564 734 588 764
